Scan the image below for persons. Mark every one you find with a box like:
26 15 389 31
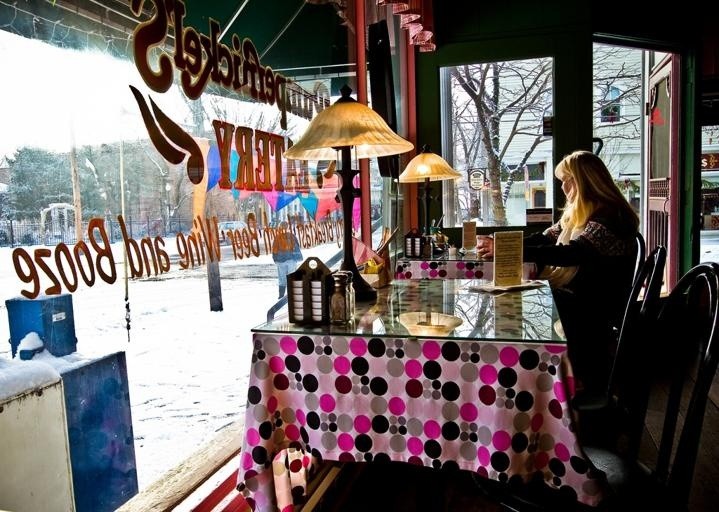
272 221 304 298
475 150 640 406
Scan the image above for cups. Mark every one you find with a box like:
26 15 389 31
449 248 457 256
476 235 493 260
710 213 719 229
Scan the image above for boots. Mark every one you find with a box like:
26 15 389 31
279 286 286 301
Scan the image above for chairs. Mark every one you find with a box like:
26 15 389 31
574 241 717 510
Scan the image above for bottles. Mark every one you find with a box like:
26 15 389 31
329 270 356 326
405 226 449 258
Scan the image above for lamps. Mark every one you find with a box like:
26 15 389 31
284 81 462 303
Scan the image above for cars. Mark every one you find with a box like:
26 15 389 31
215 221 258 247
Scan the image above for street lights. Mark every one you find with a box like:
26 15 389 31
165 181 172 234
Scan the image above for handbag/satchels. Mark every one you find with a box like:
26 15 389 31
292 246 304 261
